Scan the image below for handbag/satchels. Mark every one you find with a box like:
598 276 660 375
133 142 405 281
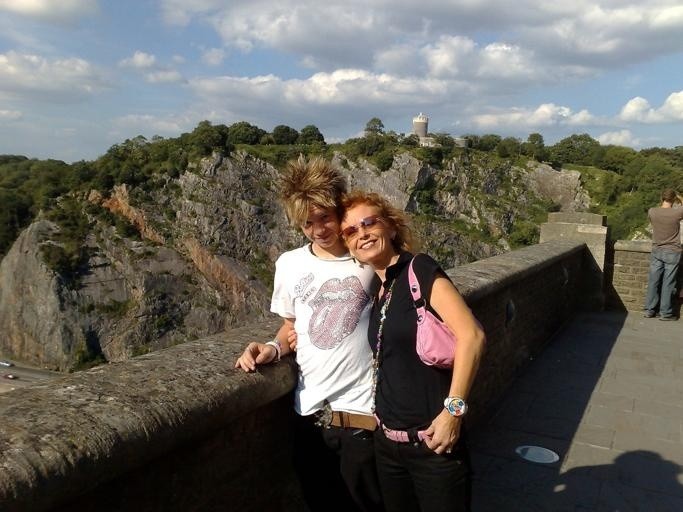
407 252 486 370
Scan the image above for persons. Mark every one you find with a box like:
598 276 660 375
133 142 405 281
642 189 682 320
233 155 387 511
339 190 486 511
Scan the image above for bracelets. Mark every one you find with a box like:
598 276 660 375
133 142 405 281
263 341 280 363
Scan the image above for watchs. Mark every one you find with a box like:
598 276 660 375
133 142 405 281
444 396 469 417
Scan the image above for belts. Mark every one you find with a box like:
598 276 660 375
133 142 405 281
319 410 377 431
374 414 430 441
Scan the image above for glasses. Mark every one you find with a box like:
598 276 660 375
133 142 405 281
339 215 385 243
